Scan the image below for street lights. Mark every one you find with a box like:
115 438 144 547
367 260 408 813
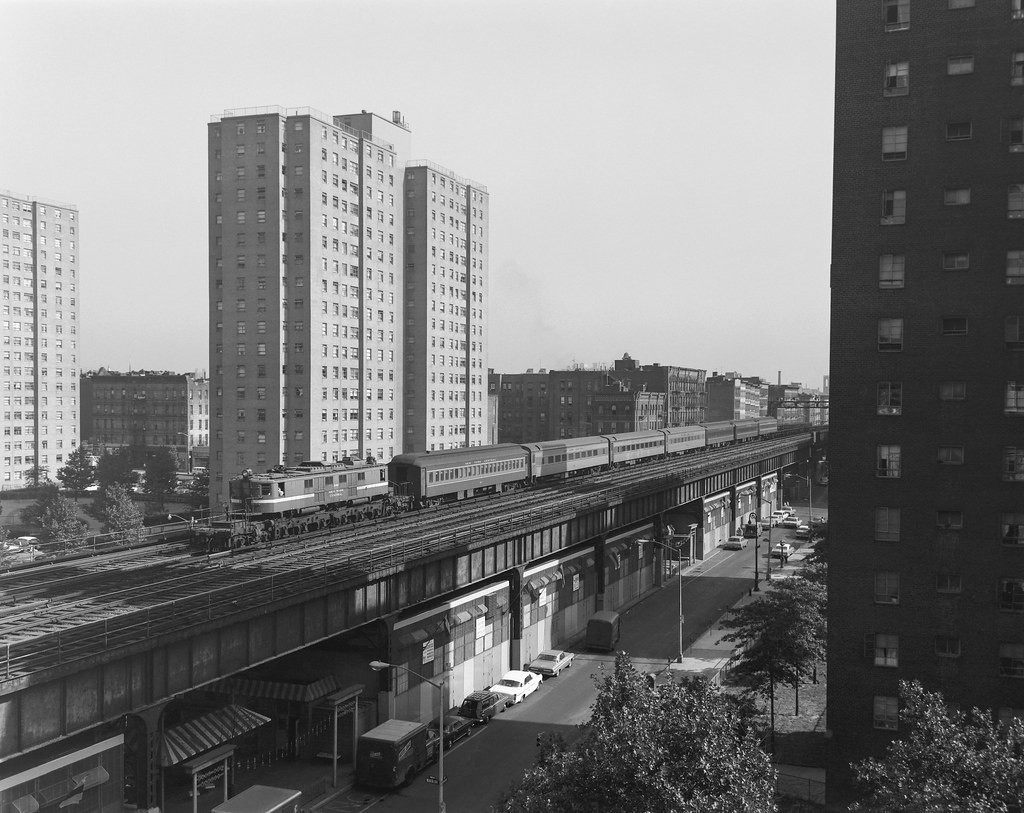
740 492 773 580
369 660 447 813
749 513 761 592
785 473 813 543
637 539 683 663
168 512 194 531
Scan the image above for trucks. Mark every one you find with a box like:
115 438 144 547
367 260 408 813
212 785 303 813
586 610 624 652
357 719 438 789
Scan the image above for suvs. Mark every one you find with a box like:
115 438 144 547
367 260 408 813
457 691 512 725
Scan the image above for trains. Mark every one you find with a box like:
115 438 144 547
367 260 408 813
229 415 777 522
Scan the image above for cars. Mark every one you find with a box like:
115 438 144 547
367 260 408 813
763 506 796 530
772 543 795 557
744 523 764 538
795 525 811 538
488 669 544 705
426 714 474 748
528 649 576 678
726 535 748 550
784 517 803 527
